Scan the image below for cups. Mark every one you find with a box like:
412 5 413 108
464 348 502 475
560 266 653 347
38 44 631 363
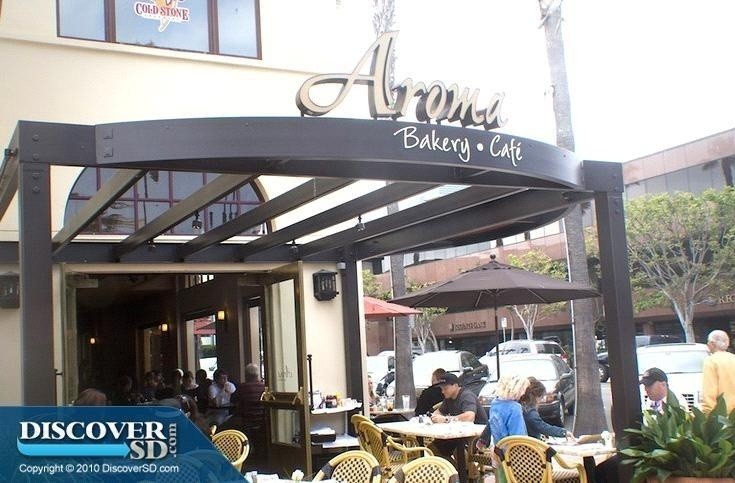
379 396 394 411
402 395 411 410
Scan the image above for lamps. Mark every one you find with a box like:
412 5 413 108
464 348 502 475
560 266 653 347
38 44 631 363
162 323 170 337
290 239 298 254
217 310 227 324
356 215 365 231
144 239 156 249
192 212 202 230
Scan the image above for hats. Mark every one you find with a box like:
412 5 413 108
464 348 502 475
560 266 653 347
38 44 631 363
434 373 458 387
637 368 668 386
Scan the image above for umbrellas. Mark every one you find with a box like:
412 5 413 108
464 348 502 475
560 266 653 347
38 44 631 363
364 295 421 320
388 254 600 379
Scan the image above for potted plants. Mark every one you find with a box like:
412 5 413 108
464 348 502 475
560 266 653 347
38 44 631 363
617 387 735 483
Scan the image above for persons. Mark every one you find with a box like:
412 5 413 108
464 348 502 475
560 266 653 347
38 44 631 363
69 363 268 460
364 357 571 471
639 368 690 417
701 329 735 417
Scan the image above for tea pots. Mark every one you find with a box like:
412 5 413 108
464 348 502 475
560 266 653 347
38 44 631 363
308 390 323 409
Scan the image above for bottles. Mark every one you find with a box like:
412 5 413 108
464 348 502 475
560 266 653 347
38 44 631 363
325 395 342 408
418 413 427 424
